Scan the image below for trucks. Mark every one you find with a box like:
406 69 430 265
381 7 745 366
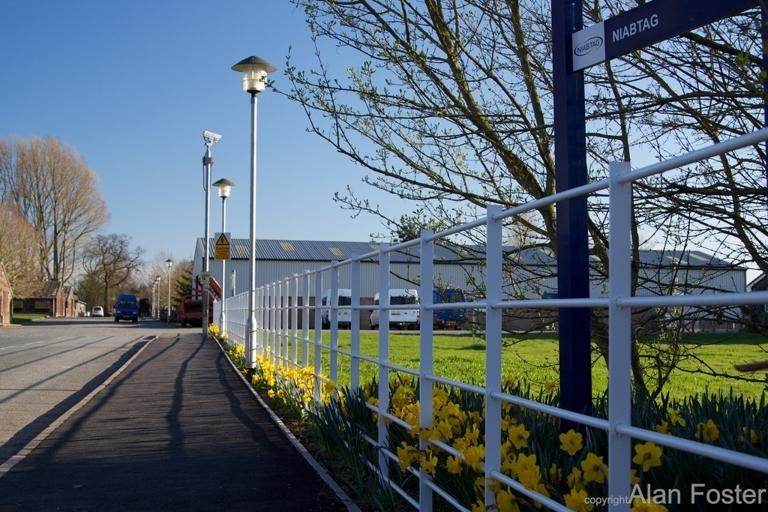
178 275 223 327
114 295 139 324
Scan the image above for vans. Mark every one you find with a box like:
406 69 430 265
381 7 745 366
322 287 351 328
434 286 467 328
92 305 105 317
365 288 421 329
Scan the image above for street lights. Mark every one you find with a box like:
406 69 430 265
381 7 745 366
214 176 235 336
166 259 173 320
231 56 280 373
151 275 161 320
